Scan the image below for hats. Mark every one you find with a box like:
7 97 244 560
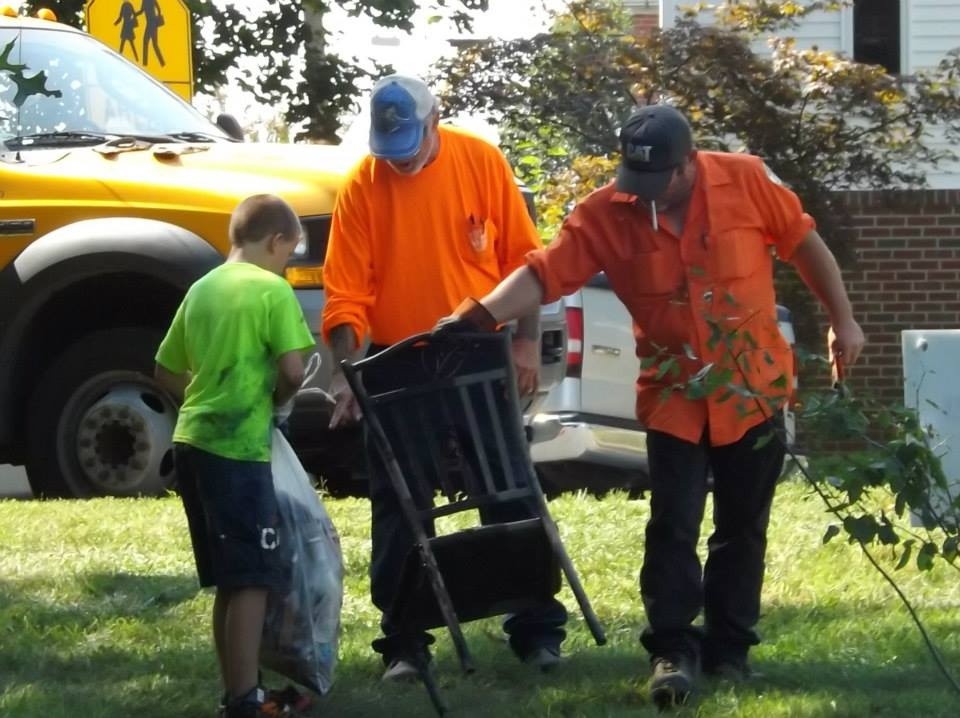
369 76 435 158
615 104 692 200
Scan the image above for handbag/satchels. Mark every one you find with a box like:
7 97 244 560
257 426 344 697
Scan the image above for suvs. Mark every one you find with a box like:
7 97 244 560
0 4 568 500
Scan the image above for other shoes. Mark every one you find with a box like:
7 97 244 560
649 651 695 710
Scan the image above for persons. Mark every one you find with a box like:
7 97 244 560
317 72 567 681
155 194 316 718
429 105 865 702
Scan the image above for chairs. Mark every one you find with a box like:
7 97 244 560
337 324 610 718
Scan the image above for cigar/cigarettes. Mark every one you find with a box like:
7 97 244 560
651 199 657 230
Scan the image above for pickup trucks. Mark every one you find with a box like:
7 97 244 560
519 270 799 501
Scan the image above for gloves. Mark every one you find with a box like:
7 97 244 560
272 396 295 425
421 297 498 377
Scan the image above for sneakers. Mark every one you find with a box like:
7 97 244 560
218 682 313 718
709 663 775 689
523 644 563 674
383 656 422 684
